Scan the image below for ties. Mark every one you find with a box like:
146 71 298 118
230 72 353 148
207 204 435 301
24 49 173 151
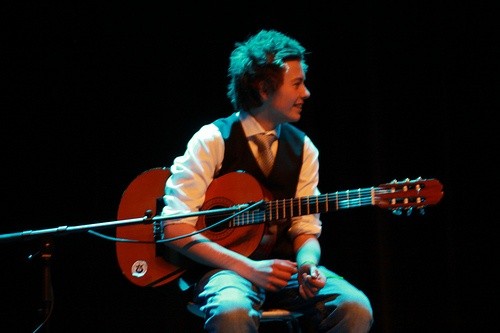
253 133 276 175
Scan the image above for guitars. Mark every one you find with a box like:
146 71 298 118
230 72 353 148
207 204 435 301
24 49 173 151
115 168 443 288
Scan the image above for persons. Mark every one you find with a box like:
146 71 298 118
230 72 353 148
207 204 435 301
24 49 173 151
161 30 373 332
